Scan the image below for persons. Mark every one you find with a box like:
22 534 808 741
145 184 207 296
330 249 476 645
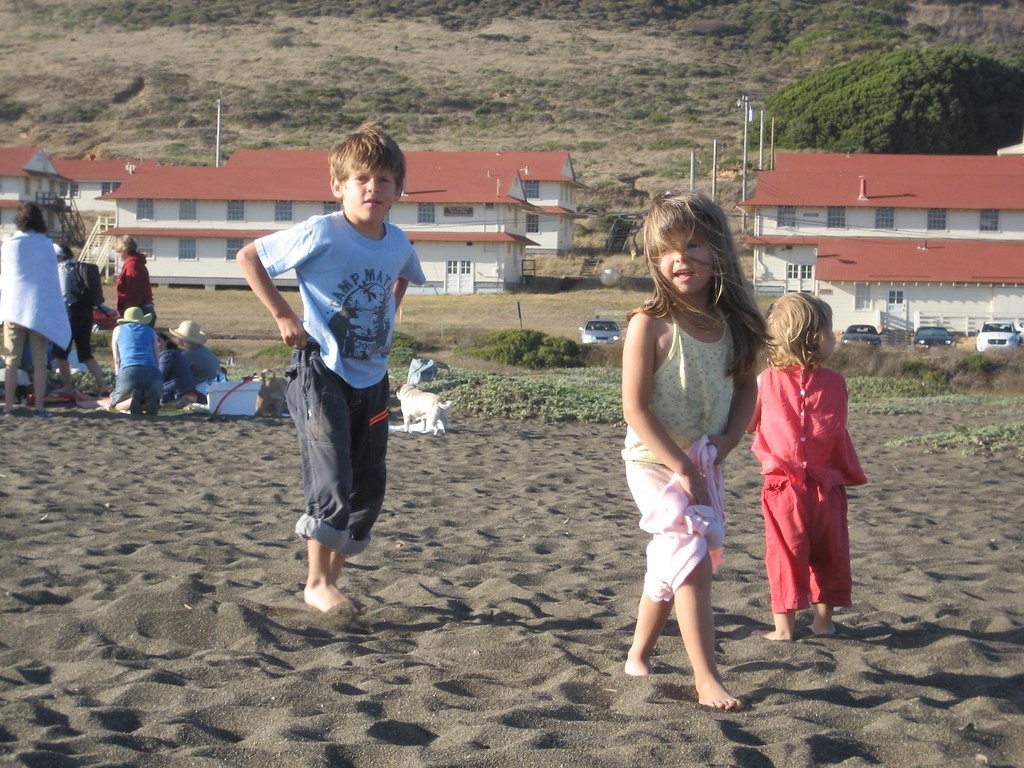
746 293 866 641
622 194 759 710
114 235 157 327
236 123 425 616
109 307 162 416
53 242 107 399
0 202 71 417
74 322 223 408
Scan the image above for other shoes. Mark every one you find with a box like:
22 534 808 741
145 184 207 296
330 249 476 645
130 389 143 415
143 390 158 416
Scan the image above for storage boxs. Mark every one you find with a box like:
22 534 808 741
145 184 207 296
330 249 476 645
205 381 262 416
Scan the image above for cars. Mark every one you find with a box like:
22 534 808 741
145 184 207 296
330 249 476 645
910 326 953 350
974 317 1024 353
840 324 883 346
578 319 623 345
92 303 118 330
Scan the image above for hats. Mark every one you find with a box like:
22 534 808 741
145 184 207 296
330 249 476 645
169 320 207 344
116 307 152 325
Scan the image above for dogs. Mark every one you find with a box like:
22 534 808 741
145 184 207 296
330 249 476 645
256 369 290 417
396 384 454 436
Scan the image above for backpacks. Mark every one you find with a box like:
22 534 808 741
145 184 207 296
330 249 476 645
64 261 105 305
